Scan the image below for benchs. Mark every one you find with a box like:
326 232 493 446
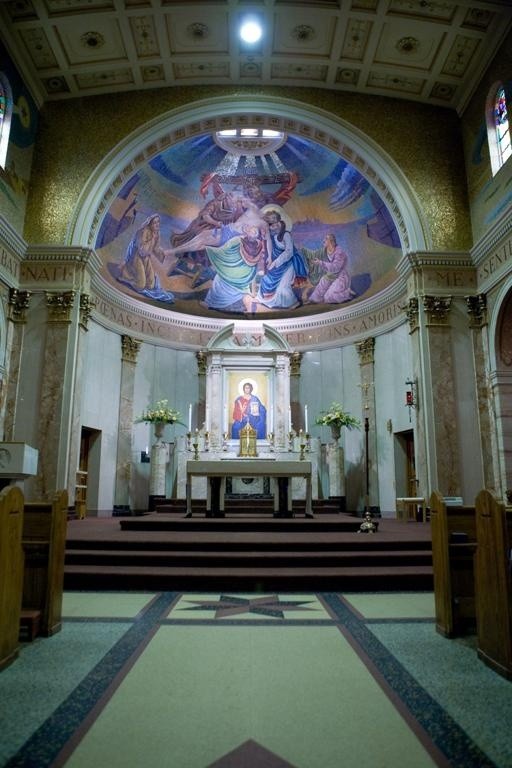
0 485 23 670
428 487 477 639
474 485 511 679
21 487 70 643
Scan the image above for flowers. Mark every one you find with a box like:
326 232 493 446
312 401 363 433
132 396 185 428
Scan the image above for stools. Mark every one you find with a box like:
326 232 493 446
394 497 427 525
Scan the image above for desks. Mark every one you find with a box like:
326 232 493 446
184 458 317 522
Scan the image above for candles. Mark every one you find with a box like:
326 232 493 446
206 407 211 432
188 401 192 433
304 404 308 432
224 408 229 434
270 406 274 432
288 405 292 431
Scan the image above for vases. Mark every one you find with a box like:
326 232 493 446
150 417 168 439
329 420 343 442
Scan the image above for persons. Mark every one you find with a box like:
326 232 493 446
115 173 356 308
231 382 267 438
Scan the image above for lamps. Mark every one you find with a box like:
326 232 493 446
405 381 417 407
